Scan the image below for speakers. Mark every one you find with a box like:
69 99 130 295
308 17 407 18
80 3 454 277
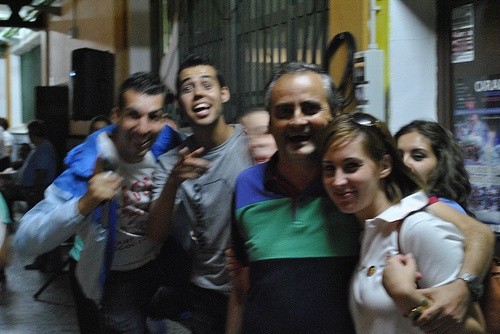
36 85 69 145
71 47 115 121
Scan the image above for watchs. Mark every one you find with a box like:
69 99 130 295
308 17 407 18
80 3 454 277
456 271 484 301
405 296 432 320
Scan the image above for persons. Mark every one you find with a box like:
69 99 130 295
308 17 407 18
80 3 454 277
0 115 180 291
226 112 487 334
149 54 255 334
224 60 496 334
12 72 189 334
393 119 471 217
